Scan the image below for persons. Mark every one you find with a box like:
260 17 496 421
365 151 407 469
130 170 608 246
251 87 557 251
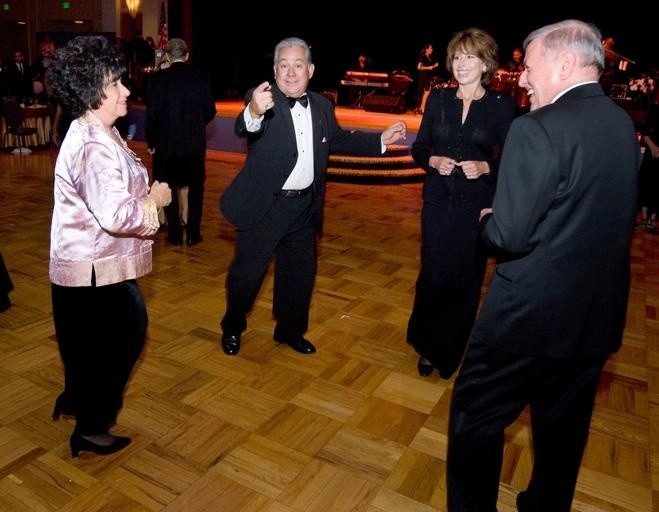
221 38 410 356
144 39 216 246
352 54 370 72
407 26 517 378
445 19 638 512
0 36 62 107
1 256 13 312
48 36 173 464
508 47 526 71
415 45 438 115
628 106 659 232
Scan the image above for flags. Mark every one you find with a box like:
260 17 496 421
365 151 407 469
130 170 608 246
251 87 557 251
157 1 167 50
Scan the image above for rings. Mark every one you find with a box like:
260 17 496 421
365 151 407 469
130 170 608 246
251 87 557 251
264 103 270 109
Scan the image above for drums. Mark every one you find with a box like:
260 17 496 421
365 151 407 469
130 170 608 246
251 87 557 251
489 68 531 107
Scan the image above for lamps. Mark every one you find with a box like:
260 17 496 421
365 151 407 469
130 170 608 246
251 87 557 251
125 0 140 36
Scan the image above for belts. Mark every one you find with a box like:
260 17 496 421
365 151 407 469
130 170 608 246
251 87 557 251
281 184 313 197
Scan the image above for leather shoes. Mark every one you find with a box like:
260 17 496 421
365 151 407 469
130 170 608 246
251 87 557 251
168 234 203 246
273 333 316 354
221 334 241 355
418 357 434 377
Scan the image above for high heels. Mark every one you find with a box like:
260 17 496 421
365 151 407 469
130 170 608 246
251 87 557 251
52 397 76 421
69 433 131 458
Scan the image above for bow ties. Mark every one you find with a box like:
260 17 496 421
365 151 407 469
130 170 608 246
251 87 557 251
286 95 307 109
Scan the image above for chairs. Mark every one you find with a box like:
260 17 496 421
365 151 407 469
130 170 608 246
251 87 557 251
3 100 39 159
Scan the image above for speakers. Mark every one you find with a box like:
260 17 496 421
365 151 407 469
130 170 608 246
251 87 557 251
362 95 407 114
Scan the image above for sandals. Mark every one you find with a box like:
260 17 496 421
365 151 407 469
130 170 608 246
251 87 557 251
640 217 656 229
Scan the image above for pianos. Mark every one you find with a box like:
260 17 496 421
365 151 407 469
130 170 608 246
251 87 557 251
341 71 389 88
